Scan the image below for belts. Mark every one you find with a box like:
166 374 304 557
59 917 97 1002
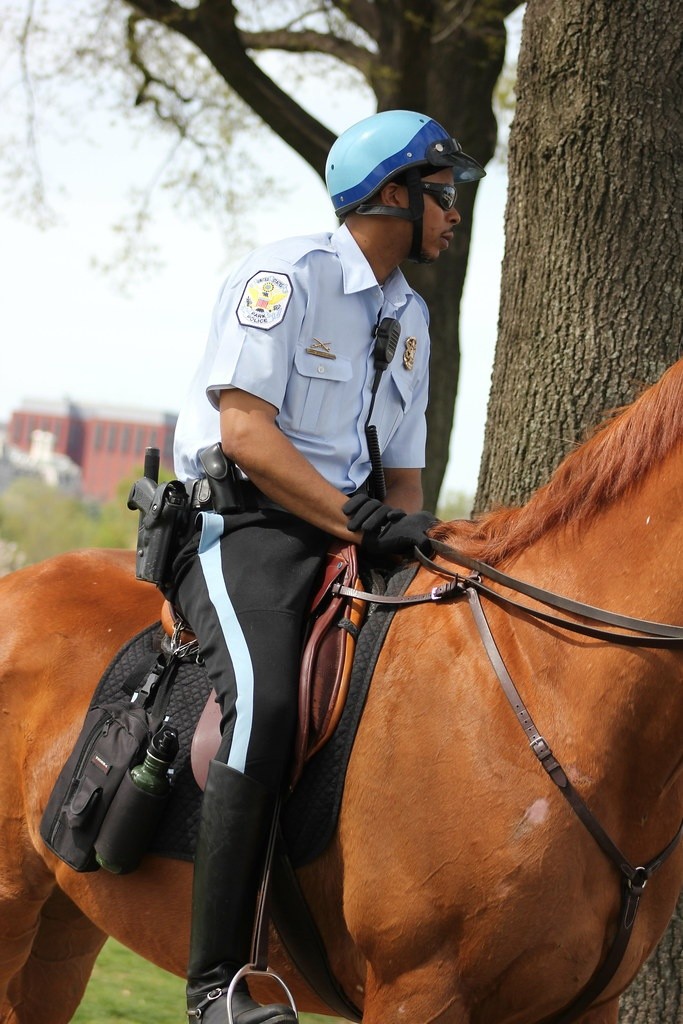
191 481 290 513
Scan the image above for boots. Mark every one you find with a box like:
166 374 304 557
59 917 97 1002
186 760 298 1024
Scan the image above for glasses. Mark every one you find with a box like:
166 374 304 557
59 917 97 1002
421 181 457 211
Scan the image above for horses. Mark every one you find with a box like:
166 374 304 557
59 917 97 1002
0 352 682 1024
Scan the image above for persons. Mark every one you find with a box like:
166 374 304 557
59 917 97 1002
157 109 486 1024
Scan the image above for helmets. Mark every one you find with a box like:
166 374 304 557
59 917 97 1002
325 110 486 220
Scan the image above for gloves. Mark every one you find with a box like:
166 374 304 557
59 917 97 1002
360 510 443 563
342 493 406 535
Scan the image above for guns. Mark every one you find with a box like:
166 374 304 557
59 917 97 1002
125 476 190 515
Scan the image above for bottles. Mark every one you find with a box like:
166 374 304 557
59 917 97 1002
96 726 179 873
